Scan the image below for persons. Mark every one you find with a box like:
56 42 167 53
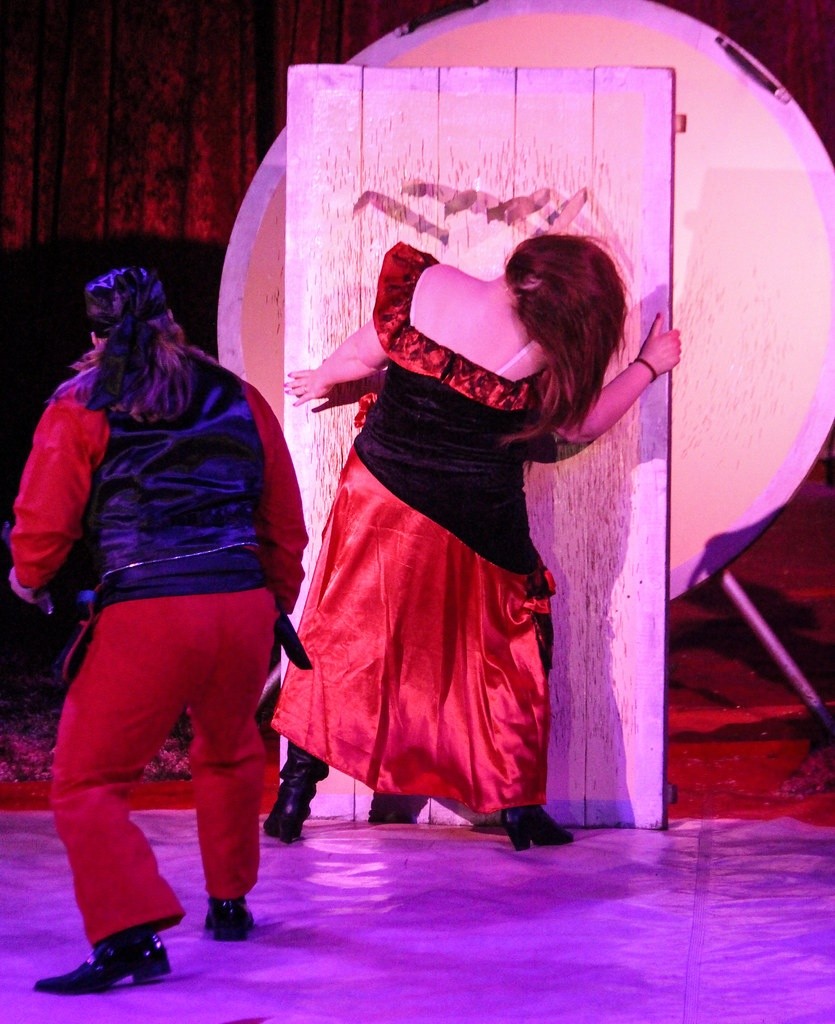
260 231 681 850
6 269 306 992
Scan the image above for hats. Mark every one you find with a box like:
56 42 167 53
85 267 171 335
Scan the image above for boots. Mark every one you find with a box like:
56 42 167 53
264 740 329 843
501 805 573 850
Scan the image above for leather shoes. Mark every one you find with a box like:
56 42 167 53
34 924 171 993
205 896 253 941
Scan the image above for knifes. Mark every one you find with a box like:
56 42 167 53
281 616 313 670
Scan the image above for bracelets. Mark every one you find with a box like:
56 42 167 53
634 357 657 384
